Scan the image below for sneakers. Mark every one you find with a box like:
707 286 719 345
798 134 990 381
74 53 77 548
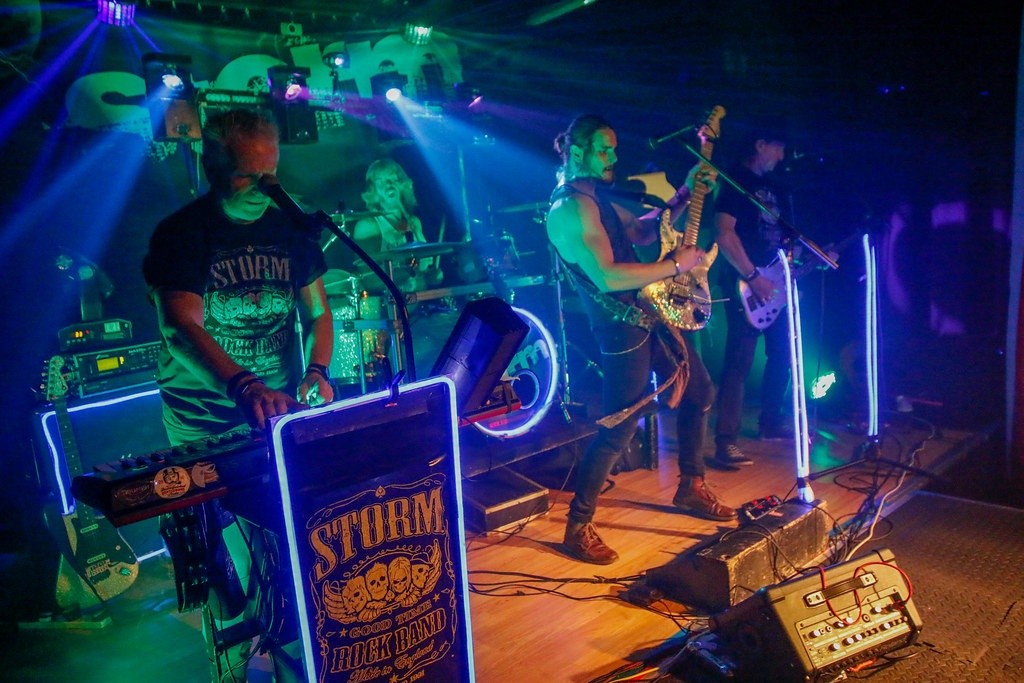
761 424 813 440
715 443 754 466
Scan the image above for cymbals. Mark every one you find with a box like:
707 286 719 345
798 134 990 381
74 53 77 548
327 211 395 224
352 240 466 267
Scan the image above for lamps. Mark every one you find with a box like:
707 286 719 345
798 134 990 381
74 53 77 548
267 64 305 102
372 70 404 100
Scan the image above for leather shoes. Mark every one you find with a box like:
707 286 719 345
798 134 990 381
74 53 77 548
564 521 616 564
672 472 739 520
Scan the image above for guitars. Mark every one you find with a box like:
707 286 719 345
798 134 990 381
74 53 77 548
734 224 874 332
639 104 728 331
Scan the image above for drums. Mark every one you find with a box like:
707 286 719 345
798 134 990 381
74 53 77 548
492 200 565 289
454 230 522 281
392 303 561 440
301 290 394 386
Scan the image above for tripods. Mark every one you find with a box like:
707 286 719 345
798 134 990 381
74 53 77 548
808 234 939 479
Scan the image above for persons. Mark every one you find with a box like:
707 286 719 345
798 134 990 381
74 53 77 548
142 109 332 683
348 157 442 290
548 114 740 563
714 118 839 467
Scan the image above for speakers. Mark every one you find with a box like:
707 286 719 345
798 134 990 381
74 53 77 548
676 500 823 613
708 547 924 683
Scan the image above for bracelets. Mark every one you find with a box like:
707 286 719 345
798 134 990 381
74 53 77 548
663 257 682 276
307 367 328 380
226 371 258 393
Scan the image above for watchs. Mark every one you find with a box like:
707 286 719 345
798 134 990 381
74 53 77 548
306 364 330 380
745 269 759 282
237 379 263 399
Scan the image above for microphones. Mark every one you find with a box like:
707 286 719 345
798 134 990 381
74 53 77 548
644 122 698 152
789 148 807 160
258 173 323 240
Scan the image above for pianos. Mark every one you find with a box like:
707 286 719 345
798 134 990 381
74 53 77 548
68 412 284 683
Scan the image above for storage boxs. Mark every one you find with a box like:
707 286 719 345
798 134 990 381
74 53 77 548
463 464 548 537
